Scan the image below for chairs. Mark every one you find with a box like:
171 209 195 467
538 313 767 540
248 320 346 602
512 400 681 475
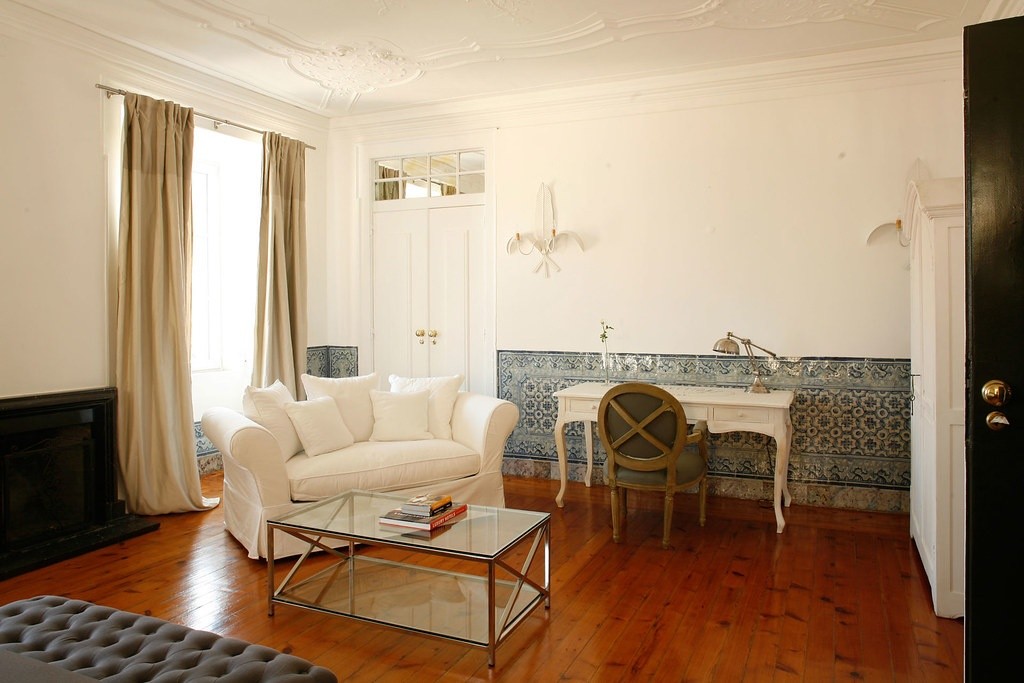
598 383 709 550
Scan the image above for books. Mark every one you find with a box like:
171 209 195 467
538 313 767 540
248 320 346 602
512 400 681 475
378 502 467 530
401 493 452 517
379 512 467 540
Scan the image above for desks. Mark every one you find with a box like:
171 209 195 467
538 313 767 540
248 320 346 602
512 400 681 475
552 382 799 531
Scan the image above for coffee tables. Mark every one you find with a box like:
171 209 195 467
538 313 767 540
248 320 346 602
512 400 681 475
267 490 553 669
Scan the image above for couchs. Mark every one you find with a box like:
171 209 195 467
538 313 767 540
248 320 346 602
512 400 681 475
201 391 518 561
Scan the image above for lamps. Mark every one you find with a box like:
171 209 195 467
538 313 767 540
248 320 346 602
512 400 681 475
712 332 779 393
505 182 584 277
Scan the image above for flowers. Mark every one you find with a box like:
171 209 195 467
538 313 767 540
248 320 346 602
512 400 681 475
599 319 614 342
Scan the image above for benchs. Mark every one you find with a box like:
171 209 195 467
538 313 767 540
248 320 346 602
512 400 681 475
0 594 339 683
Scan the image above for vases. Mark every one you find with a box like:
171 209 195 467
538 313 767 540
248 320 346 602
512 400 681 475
601 343 611 383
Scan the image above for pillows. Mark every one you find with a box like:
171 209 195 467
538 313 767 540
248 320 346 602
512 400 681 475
301 373 380 442
369 390 433 442
284 397 353 456
389 374 465 441
242 379 304 462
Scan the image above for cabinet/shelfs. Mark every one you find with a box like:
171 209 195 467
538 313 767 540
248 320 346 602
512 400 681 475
900 176 966 620
